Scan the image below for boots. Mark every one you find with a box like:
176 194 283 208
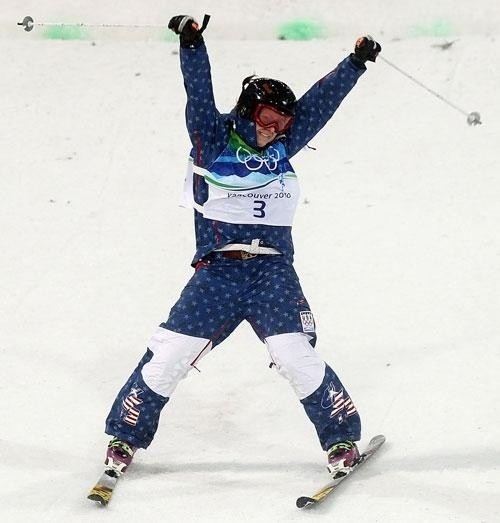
104 436 137 479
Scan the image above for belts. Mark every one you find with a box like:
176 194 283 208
208 251 257 260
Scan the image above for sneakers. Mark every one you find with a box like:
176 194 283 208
326 441 360 480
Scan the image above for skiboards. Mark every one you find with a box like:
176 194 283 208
86 435 385 510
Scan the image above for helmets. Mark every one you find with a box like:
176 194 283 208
237 77 297 133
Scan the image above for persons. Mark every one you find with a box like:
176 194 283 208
104 14 379 478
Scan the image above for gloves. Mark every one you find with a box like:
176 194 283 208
355 38 382 63
169 14 203 44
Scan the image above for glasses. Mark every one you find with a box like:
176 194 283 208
254 104 293 133
